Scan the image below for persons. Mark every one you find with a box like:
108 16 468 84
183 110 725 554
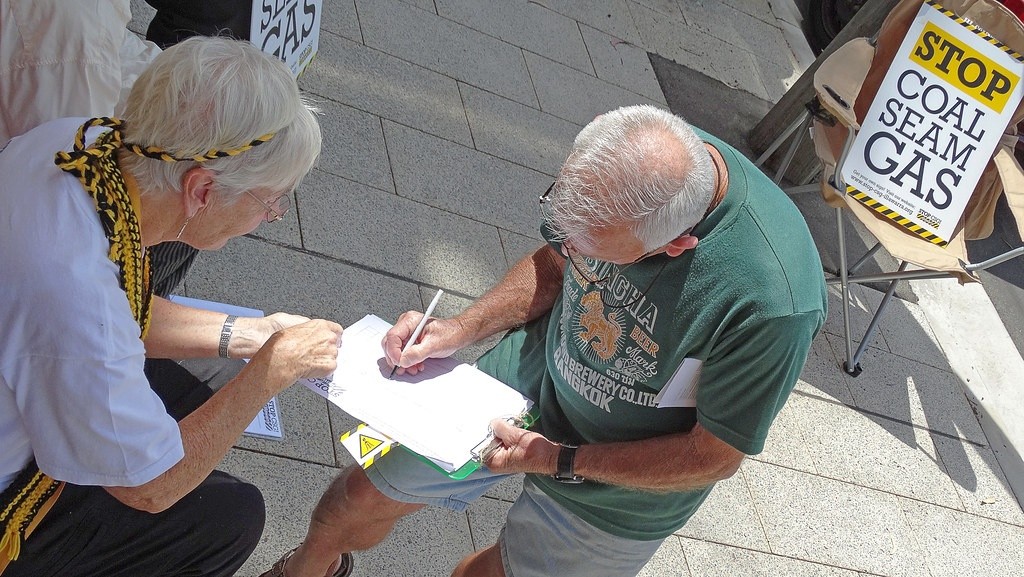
0 36 345 577
255 106 828 577
0 0 201 300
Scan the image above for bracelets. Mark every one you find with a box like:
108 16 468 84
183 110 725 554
218 315 239 359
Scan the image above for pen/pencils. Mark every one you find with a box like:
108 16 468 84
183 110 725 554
388 289 445 380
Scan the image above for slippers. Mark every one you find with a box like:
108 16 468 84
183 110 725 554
258 542 354 577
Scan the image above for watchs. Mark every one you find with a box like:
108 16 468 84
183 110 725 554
553 439 585 485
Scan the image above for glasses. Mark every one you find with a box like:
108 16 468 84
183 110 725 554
538 181 694 288
244 190 294 224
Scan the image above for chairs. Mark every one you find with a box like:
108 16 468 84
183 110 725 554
746 0 1024 373
